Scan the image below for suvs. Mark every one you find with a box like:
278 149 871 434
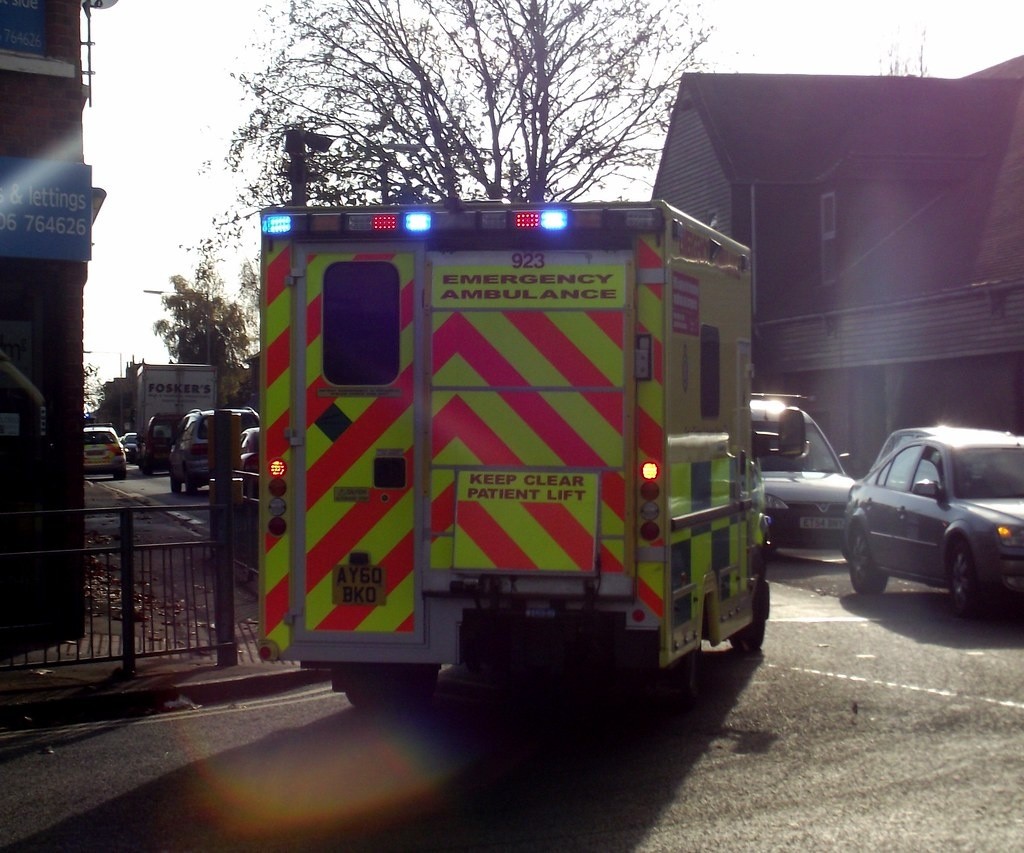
169 406 260 496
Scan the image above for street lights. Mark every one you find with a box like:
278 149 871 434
144 291 210 363
83 351 123 435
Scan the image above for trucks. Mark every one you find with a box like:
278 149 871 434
750 399 859 548
131 364 219 472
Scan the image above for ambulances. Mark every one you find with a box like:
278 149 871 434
258 198 809 723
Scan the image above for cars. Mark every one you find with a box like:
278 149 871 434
841 435 1024 622
869 427 1010 469
85 427 127 480
141 413 186 477
121 432 138 461
239 427 261 500
